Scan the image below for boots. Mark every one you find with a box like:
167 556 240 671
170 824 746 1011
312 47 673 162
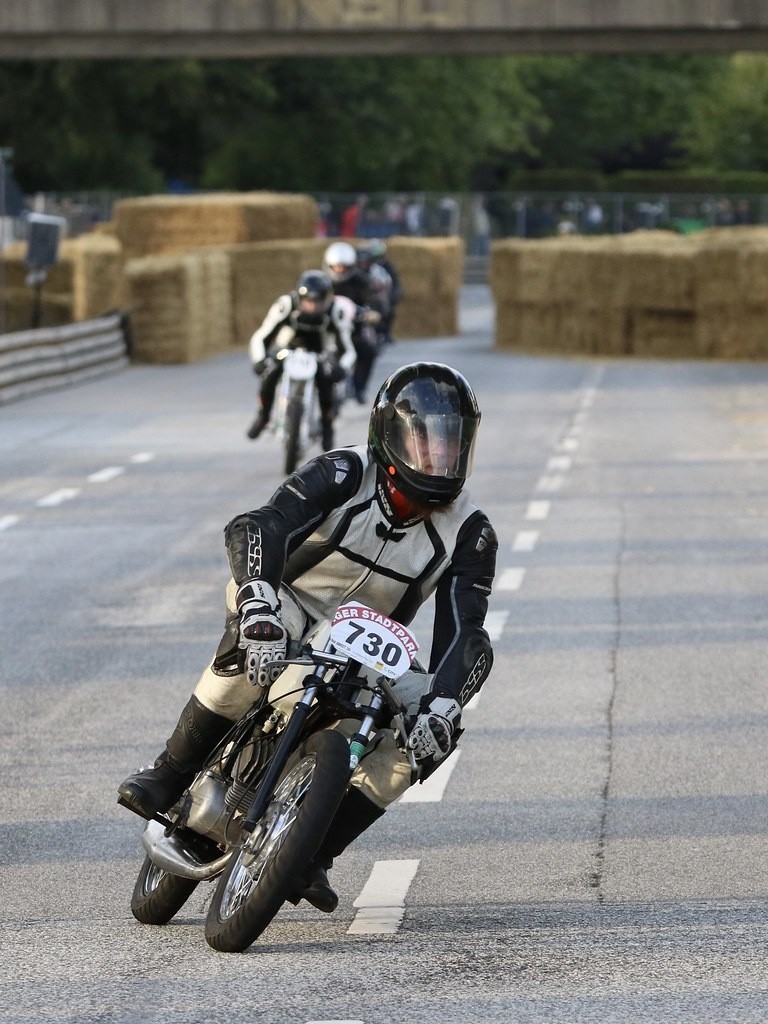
297 782 387 913
118 693 238 817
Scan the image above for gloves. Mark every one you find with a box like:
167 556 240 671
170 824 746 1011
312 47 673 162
235 578 287 686
396 693 465 781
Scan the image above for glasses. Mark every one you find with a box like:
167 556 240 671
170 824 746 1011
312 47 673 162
406 427 470 458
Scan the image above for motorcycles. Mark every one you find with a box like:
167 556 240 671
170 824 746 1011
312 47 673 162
324 291 396 421
116 615 462 953
253 346 341 478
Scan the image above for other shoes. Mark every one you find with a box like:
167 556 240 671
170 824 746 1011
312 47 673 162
319 423 333 451
249 414 268 437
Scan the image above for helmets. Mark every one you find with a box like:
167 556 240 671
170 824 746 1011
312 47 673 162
323 242 358 283
367 360 481 506
295 271 333 314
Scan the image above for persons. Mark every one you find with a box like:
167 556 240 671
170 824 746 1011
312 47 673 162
249 238 400 452
118 362 497 913
19 195 751 239
